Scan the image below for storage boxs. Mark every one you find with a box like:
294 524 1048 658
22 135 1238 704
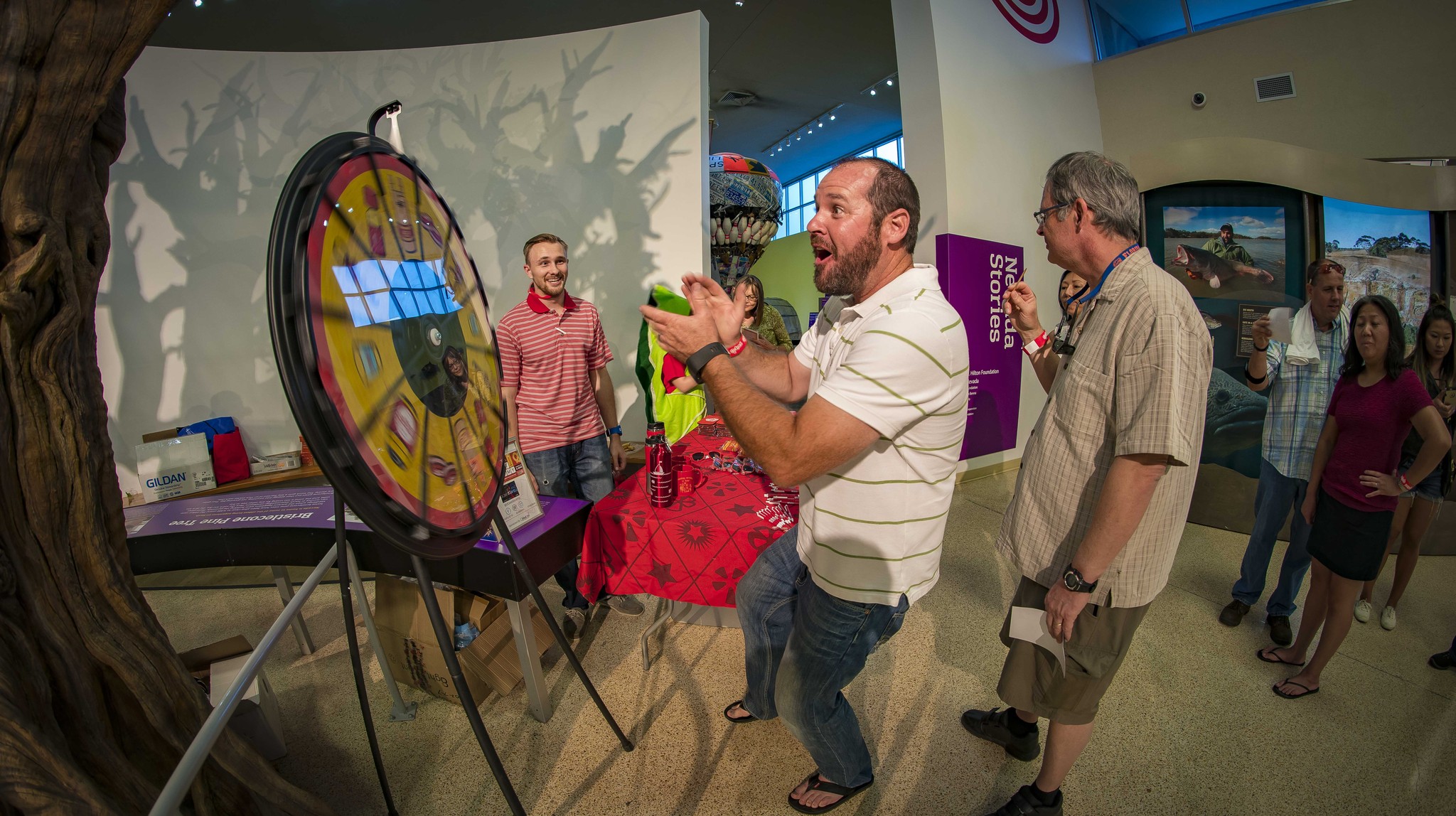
250 450 302 476
135 427 216 502
449 586 505 633
372 572 553 708
176 634 287 760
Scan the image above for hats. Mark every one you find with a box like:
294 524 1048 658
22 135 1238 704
1220 223 1234 234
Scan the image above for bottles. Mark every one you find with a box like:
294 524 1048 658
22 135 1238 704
647 421 665 438
644 435 656 496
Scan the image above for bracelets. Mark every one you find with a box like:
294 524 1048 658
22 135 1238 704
1253 341 1270 352
1400 473 1414 490
776 346 778 351
727 333 747 356
1021 329 1050 355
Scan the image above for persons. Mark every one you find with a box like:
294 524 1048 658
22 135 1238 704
442 346 469 417
958 151 1214 816
642 156 967 816
651 274 792 445
495 231 645 641
1184 223 1254 280
1219 258 1456 698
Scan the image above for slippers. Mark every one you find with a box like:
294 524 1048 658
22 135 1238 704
1257 648 1306 666
722 700 758 722
1273 678 1319 699
787 773 875 815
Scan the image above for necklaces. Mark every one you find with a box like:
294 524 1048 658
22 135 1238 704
1431 368 1437 373
1434 378 1439 381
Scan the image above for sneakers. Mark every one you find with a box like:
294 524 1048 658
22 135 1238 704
984 785 1064 816
961 708 1041 761
599 594 645 616
1353 598 1371 623
1219 600 1251 627
562 606 588 641
1267 611 1293 644
1381 606 1396 630
1430 650 1456 670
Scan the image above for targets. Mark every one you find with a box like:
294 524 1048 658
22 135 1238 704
266 132 509 562
992 0 1060 44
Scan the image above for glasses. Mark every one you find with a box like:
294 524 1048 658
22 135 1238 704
1310 264 1346 285
1033 201 1075 226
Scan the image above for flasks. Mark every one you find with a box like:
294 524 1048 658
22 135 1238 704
650 433 673 508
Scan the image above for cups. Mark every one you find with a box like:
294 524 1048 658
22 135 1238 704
672 465 703 497
671 455 692 468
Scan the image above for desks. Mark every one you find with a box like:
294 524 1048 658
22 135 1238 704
122 412 801 721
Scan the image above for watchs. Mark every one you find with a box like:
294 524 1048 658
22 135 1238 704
686 341 730 384
1063 562 1098 593
1397 478 1408 492
606 425 622 436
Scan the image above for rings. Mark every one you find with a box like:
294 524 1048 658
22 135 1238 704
1053 622 1062 626
1443 405 1445 409
1375 482 1377 487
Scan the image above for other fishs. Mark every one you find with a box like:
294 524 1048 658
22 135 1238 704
1171 243 1273 288
